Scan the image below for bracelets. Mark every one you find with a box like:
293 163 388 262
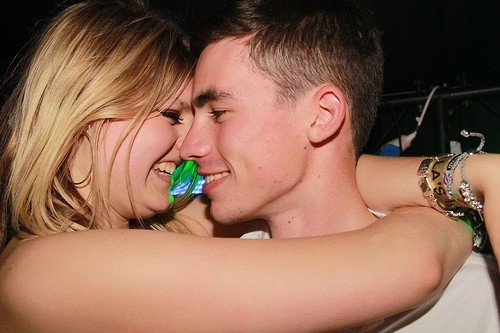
417 129 484 218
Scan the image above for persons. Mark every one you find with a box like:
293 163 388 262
0 0 500 333
177 0 500 333
